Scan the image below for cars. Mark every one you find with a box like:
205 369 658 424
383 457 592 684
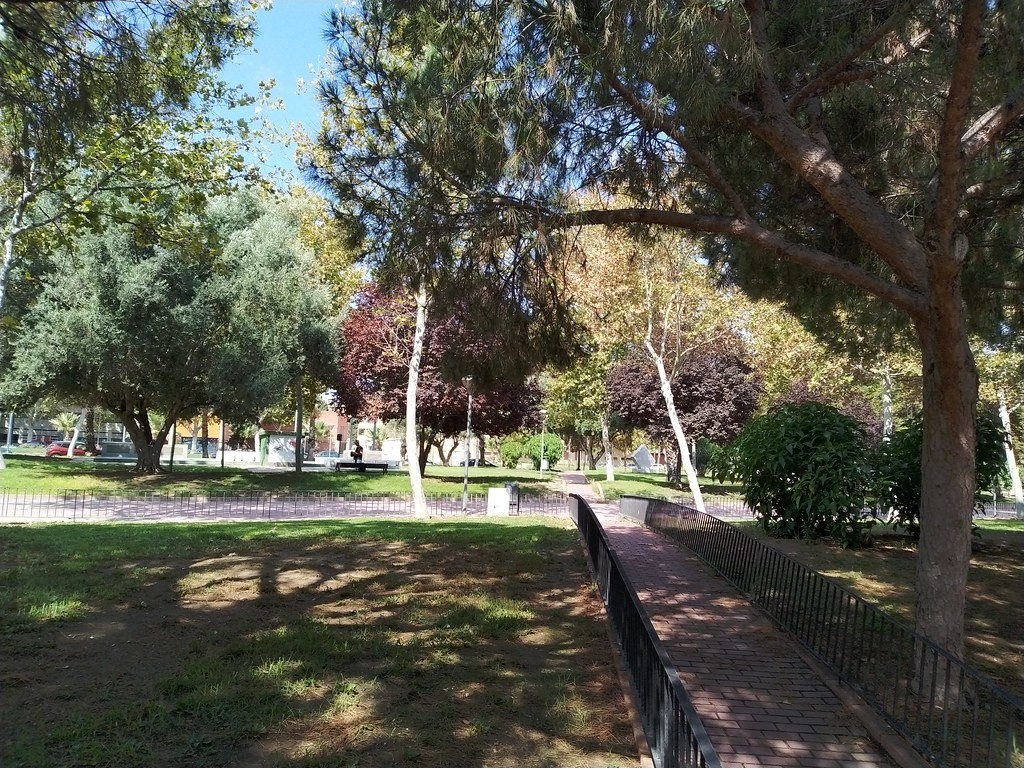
315 451 340 458
46 441 91 457
460 458 499 468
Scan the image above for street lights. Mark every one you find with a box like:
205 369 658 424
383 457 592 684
539 408 551 475
461 373 477 513
323 421 335 468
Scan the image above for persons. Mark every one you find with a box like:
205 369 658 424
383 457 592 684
350 439 363 463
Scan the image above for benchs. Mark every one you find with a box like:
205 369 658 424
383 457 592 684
336 462 389 475
